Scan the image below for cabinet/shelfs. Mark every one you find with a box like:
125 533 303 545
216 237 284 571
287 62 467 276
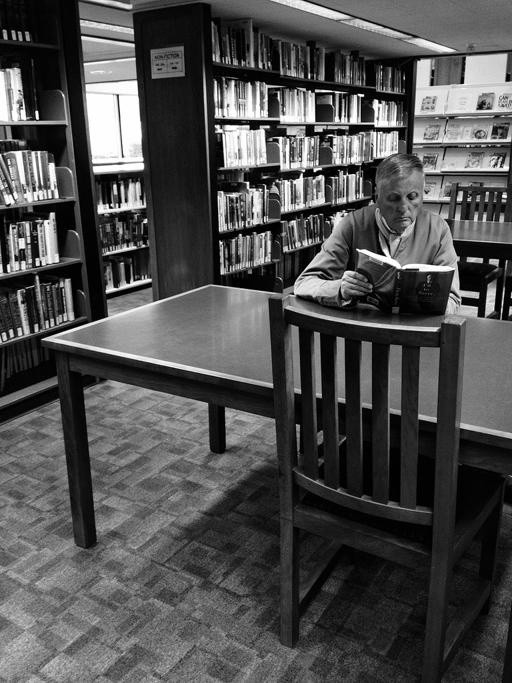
130 2 409 302
92 157 151 297
1 3 105 395
411 110 512 206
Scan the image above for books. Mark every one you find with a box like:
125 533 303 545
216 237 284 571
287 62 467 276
420 85 512 198
0 66 76 395
217 180 350 276
95 175 151 291
354 247 454 316
211 21 405 128
214 124 399 211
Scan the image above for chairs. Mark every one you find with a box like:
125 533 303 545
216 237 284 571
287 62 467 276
267 291 507 682
445 182 512 319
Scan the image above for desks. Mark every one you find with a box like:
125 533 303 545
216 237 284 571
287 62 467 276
42 282 509 548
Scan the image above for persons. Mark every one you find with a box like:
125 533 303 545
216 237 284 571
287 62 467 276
293 152 462 314
